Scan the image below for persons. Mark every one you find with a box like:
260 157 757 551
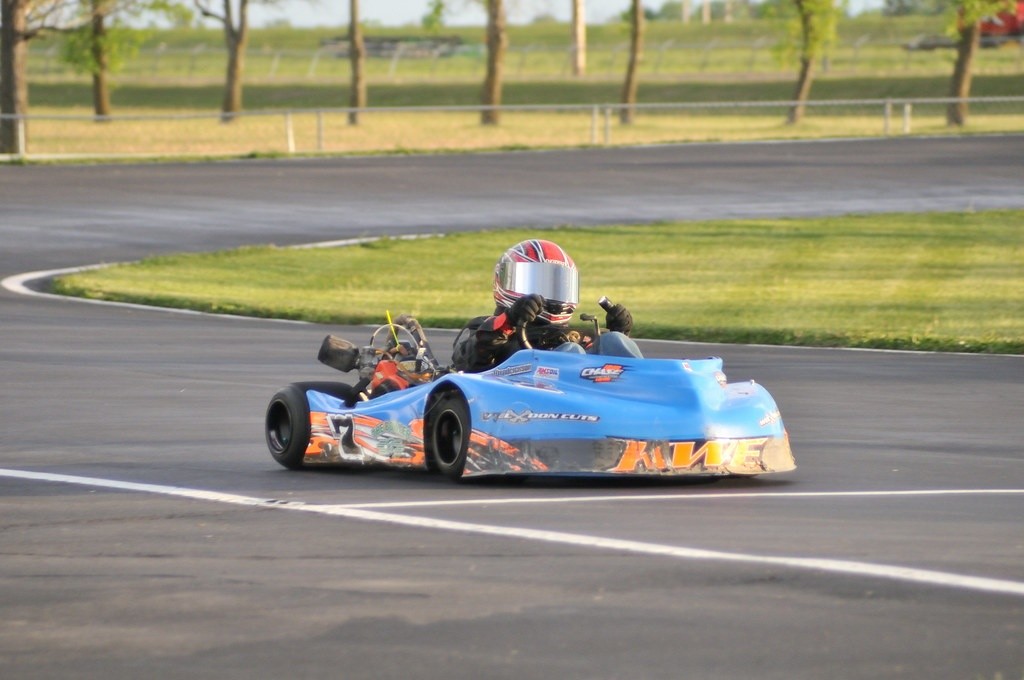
452 239 643 373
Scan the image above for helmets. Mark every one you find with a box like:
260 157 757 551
492 239 579 325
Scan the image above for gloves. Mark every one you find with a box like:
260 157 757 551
504 293 545 327
606 304 633 337
